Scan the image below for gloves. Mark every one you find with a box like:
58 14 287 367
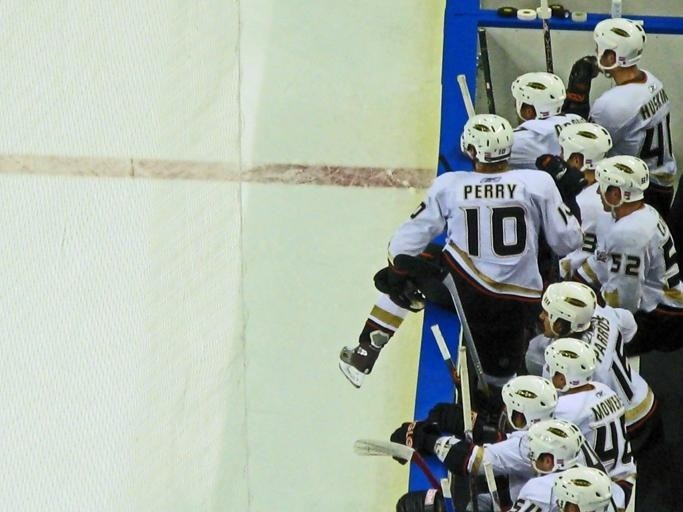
430 403 482 444
567 56 598 102
390 422 439 465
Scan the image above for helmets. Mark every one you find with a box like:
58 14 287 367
594 18 647 68
502 281 612 512
460 72 649 203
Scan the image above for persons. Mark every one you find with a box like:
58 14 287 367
339 18 683 512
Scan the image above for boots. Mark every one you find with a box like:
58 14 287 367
340 342 380 374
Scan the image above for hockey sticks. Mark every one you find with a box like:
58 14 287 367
394 254 490 396
353 438 443 496
